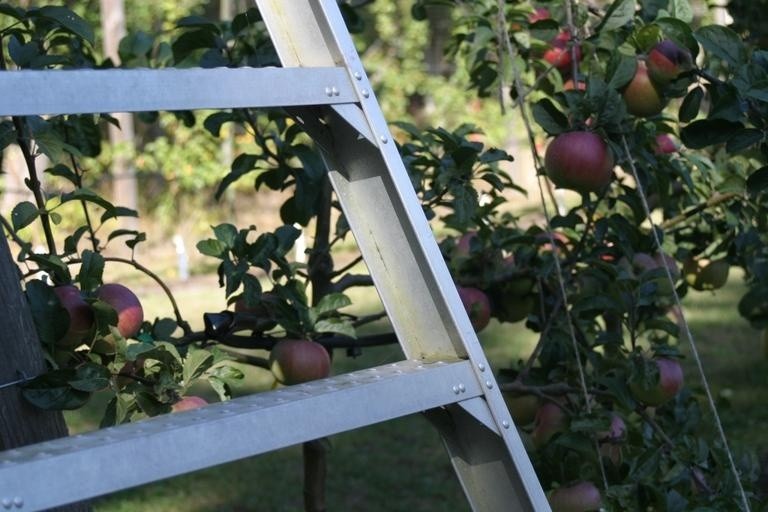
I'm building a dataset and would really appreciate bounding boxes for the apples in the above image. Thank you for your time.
[457,8,712,512]
[268,338,331,385]
[88,284,143,338]
[52,284,95,331]
[170,397,207,413]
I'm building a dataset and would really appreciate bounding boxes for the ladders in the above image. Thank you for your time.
[0,0,557,512]
